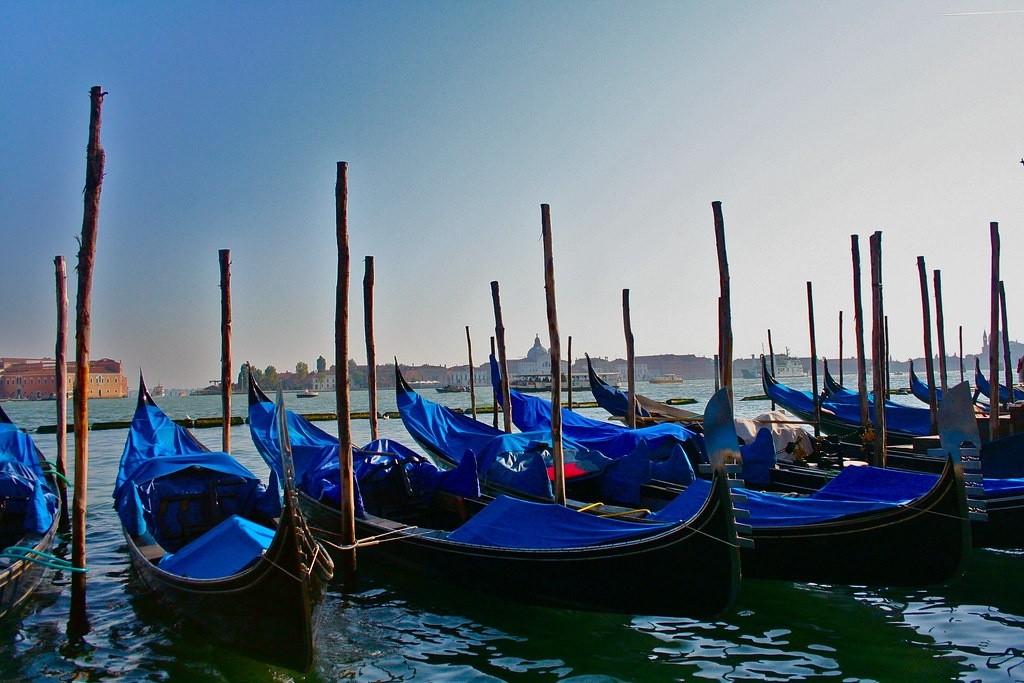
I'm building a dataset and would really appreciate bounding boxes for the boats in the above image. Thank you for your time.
[243,361,746,626]
[509,371,623,392]
[487,339,1024,555]
[393,356,970,593]
[1,404,62,628]
[435,384,471,394]
[150,385,166,398]
[112,367,339,674]
[584,348,1024,481]
[295,389,319,398]
